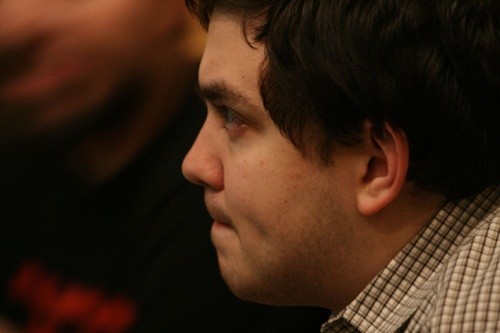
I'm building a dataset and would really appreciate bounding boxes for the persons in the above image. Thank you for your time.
[0,1,336,333]
[181,0,500,333]
